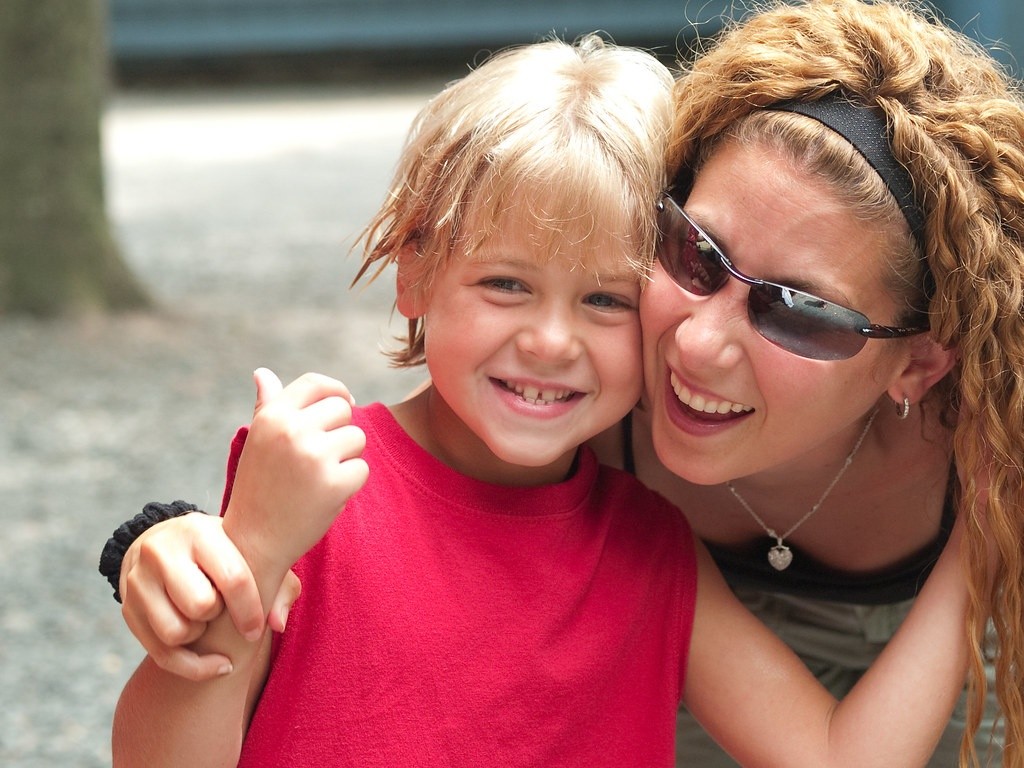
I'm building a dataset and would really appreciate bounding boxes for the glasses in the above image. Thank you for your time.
[657,171,931,360]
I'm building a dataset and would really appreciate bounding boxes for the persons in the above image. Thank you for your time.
[93,2,1024,768]
[112,28,994,767]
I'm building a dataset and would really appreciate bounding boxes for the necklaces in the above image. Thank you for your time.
[725,415,884,569]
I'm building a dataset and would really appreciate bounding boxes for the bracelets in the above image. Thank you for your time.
[98,500,207,605]
